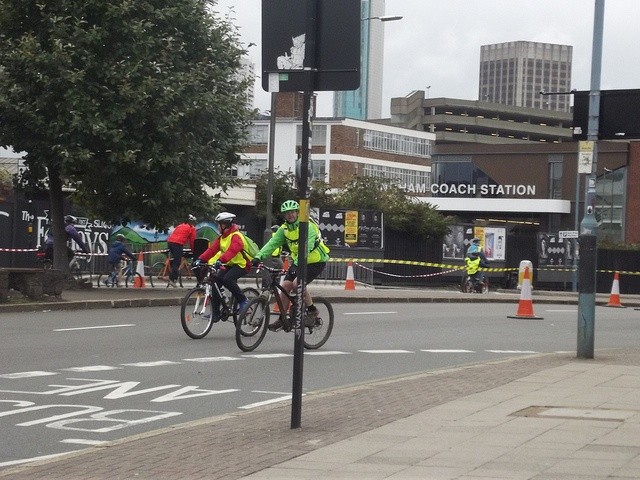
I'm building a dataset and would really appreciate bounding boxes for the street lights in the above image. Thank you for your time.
[262,16,402,290]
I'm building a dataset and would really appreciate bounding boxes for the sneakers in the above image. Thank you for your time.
[240,298,249,314]
[268,320,282,328]
[305,309,319,327]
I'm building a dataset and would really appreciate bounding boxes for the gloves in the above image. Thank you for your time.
[252,258,260,267]
[191,259,201,266]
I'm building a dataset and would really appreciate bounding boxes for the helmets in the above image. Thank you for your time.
[215,212,236,223]
[189,214,196,221]
[64,215,78,224]
[280,200,299,212]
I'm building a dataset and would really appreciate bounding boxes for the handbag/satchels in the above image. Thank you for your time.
[243,234,259,257]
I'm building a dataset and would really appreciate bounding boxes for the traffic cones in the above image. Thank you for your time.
[344,261,356,290]
[507,266,544,319]
[605,272,624,307]
[272,259,294,314]
[133,250,145,289]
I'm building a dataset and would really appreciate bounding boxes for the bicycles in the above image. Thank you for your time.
[235,259,334,352]
[97,257,143,288]
[461,261,490,294]
[181,260,264,339]
[149,250,199,289]
[35,251,91,283]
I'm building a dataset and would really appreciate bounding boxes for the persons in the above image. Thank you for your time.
[193,213,252,319]
[463,239,492,292]
[42,215,91,272]
[252,200,330,334]
[165,213,196,289]
[106,233,137,288]
